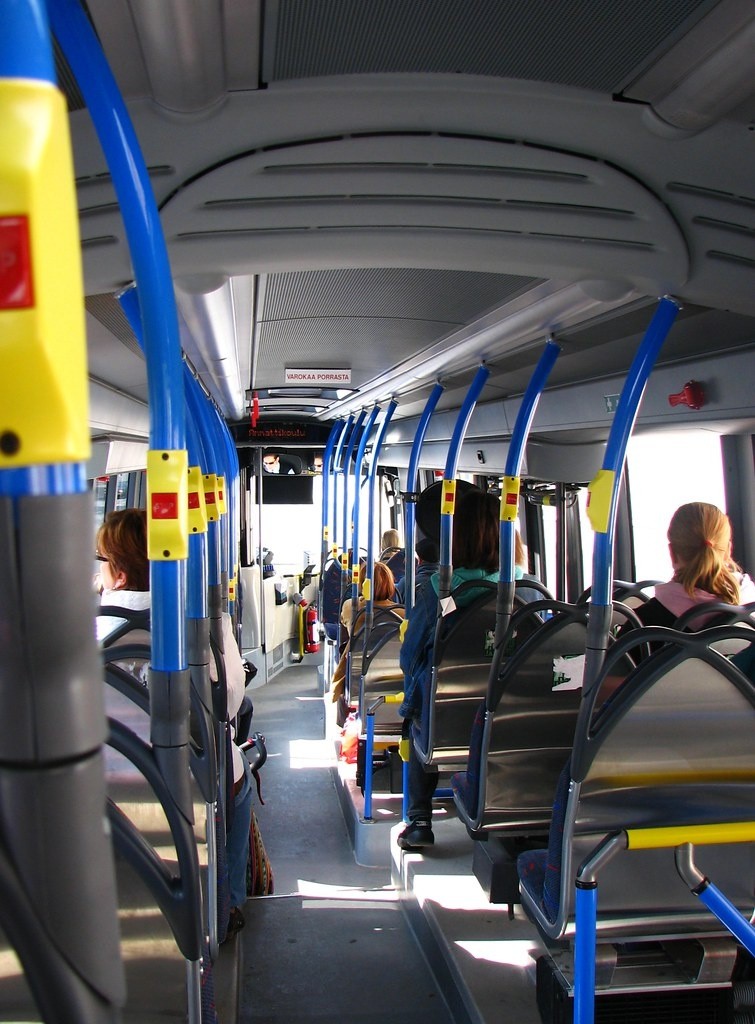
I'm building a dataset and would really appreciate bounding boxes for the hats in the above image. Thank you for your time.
[415,538,439,562]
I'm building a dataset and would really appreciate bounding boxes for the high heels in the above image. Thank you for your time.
[226,907,245,939]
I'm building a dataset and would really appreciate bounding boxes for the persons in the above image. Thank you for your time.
[595,502,755,707]
[329,481,480,703]
[95,509,253,944]
[397,494,548,850]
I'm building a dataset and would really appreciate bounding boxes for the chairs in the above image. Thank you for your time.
[315,547,755,1024]
[0,602,227,1024]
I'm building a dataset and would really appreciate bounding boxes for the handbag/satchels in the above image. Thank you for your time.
[246,806,273,897]
[242,662,257,688]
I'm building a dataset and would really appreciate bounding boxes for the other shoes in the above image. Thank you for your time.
[397,816,434,850]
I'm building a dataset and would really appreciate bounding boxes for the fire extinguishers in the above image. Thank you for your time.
[303,600,320,652]
